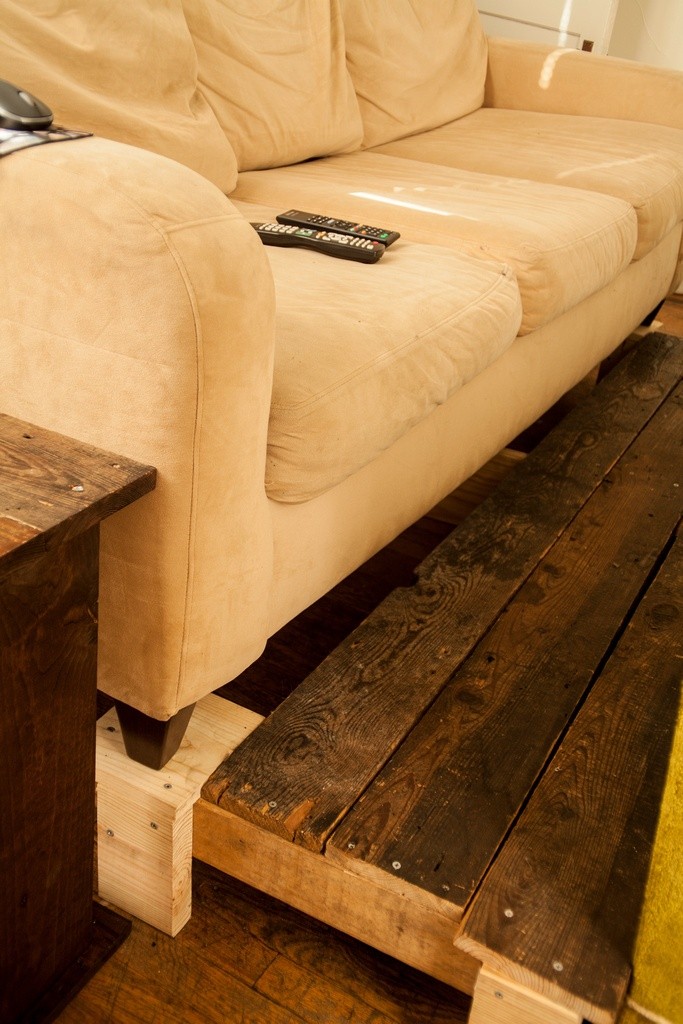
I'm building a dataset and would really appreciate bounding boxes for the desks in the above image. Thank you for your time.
[0,410,158,1024]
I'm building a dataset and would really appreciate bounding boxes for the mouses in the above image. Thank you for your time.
[0,81,54,130]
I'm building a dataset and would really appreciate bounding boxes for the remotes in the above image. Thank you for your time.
[250,210,401,265]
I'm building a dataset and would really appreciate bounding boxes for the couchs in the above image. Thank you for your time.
[0,1,683,771]
